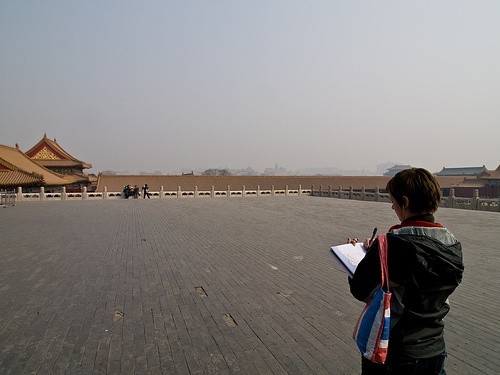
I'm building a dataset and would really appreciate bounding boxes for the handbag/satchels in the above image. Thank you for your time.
[352,233,392,365]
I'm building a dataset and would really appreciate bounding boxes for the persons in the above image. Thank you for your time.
[347,168,464,375]
[123,183,151,199]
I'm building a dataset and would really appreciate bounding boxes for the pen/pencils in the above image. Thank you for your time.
[366,227,377,251]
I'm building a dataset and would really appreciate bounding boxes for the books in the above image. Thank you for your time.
[330,242,369,279]
